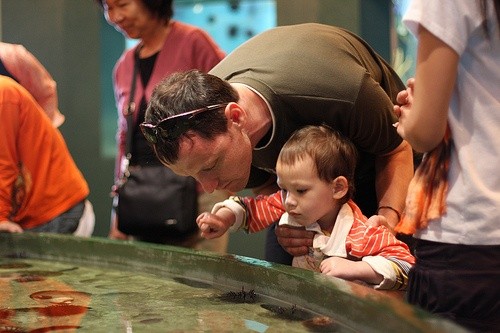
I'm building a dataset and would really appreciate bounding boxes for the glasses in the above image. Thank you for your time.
[138,103,228,143]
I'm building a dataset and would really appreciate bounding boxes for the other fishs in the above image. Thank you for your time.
[1,288,93,333]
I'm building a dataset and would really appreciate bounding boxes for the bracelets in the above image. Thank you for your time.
[377,206,401,223]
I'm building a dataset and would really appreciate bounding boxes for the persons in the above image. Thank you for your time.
[393,0,500,333]
[140,22,423,266]
[0,41,95,240]
[99,0,229,254]
[196,125,416,291]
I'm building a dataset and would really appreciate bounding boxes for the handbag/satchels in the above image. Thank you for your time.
[115,164,197,242]
